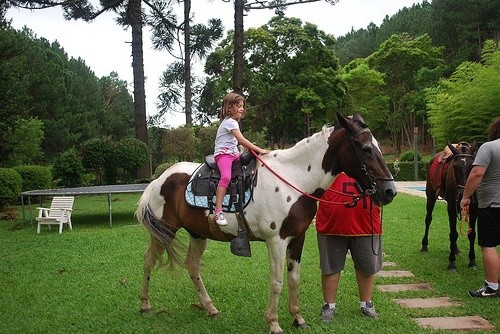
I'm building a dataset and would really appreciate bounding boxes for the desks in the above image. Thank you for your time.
[21,183,148,228]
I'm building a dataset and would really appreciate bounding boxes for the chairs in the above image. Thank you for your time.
[35,196,74,234]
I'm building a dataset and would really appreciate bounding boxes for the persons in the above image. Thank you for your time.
[460,116,500,299]
[315,171,384,323]
[214,93,270,226]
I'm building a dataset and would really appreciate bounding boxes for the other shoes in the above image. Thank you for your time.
[319,303,336,323]
[360,301,377,318]
[214,212,228,226]
[468,280,500,298]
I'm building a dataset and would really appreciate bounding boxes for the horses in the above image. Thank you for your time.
[421,139,478,272]
[134,112,397,334]
